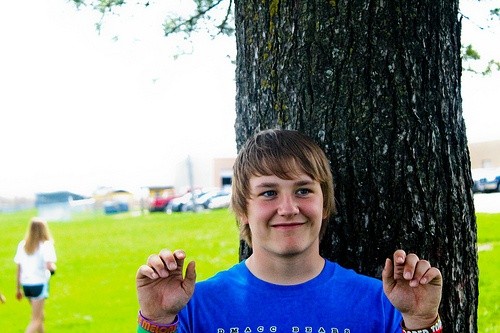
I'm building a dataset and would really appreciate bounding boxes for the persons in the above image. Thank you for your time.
[1,289,7,306]
[14,217,58,333]
[135,129,448,333]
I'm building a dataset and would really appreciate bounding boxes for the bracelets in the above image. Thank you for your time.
[400,314,443,333]
[137,326,150,333]
[139,310,181,333]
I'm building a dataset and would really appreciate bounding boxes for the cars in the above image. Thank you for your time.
[142,186,232,212]
[470,167,500,195]
